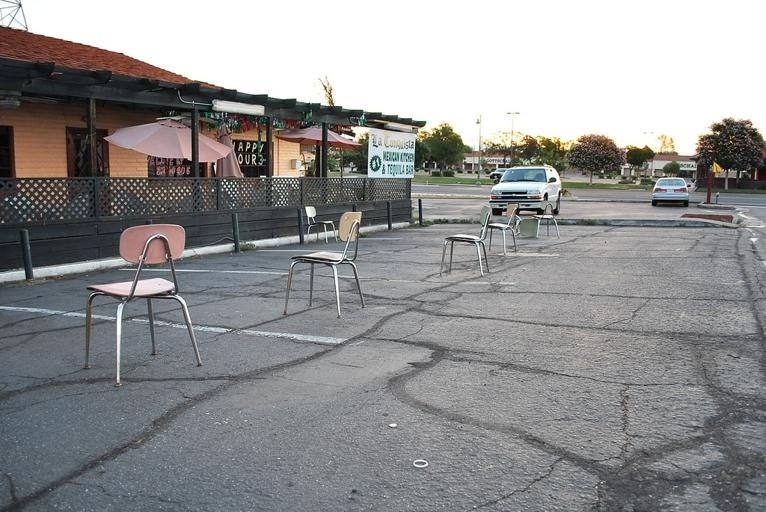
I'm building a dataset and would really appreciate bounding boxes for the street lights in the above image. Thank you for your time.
[507,112,520,163]
[476,114,482,179]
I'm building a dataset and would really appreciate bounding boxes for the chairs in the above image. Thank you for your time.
[283,205,367,318]
[87,224,201,382]
[439,201,561,278]
[511,173,544,181]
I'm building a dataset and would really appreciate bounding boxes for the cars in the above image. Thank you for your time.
[489,168,507,179]
[651,178,691,207]
[489,166,563,215]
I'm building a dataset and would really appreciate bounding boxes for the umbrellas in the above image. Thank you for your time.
[103,120,233,178]
[216,121,245,178]
[272,125,363,178]
